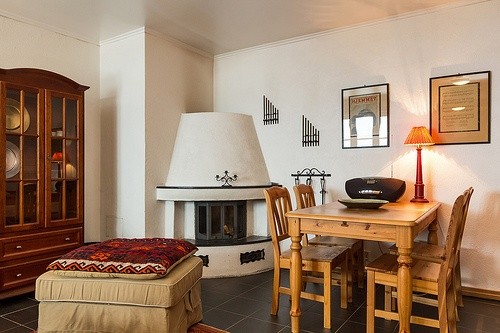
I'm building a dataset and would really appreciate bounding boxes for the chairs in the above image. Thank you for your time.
[263,185,474,333]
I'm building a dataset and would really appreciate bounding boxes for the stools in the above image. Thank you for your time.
[35,238,203,333]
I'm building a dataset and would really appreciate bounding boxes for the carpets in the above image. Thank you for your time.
[188,323,230,333]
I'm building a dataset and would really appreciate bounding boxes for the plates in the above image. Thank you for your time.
[5,97,30,134]
[6,140,20,179]
[337,198,389,209]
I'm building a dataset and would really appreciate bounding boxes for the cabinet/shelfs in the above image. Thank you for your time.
[0,67,85,300]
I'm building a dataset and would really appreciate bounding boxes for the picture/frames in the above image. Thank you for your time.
[427,70,492,146]
[342,84,395,150]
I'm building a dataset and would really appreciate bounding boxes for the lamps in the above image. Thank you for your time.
[404,126,435,203]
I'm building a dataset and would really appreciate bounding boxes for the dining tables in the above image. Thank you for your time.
[285,198,442,333]
